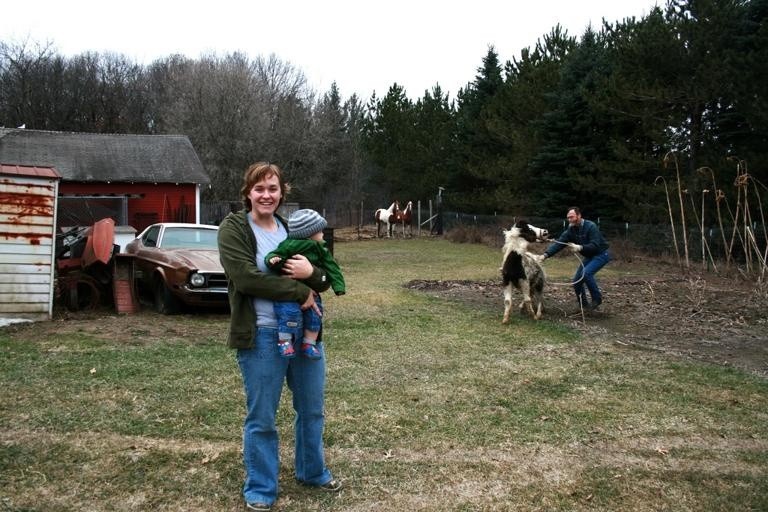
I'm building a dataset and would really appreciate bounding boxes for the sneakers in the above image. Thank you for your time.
[320,479,343,491]
[587,301,602,312]
[300,343,321,361]
[572,307,581,314]
[246,501,270,511]
[278,339,296,359]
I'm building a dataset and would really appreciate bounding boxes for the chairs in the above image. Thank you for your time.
[161,237,181,245]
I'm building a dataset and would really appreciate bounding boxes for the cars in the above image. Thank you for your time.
[125,222,233,317]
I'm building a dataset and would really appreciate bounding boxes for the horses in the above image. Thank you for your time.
[498,215,550,324]
[374,200,414,240]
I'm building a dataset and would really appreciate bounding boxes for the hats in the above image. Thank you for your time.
[287,209,327,238]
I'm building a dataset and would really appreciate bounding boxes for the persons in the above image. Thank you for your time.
[537,206,611,312]
[262,207,347,361]
[215,159,343,510]
